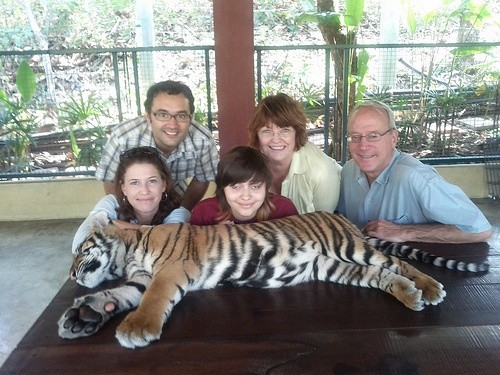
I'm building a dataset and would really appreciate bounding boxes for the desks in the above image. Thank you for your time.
[0,230,500,375]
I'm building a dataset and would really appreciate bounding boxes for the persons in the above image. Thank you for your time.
[190,145,300,226]
[94,79,220,213]
[71,146,191,255]
[334,100,493,243]
[247,93,343,217]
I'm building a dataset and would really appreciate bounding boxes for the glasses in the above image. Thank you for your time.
[120,146,164,170]
[257,127,297,139]
[148,108,192,122]
[345,129,391,143]
